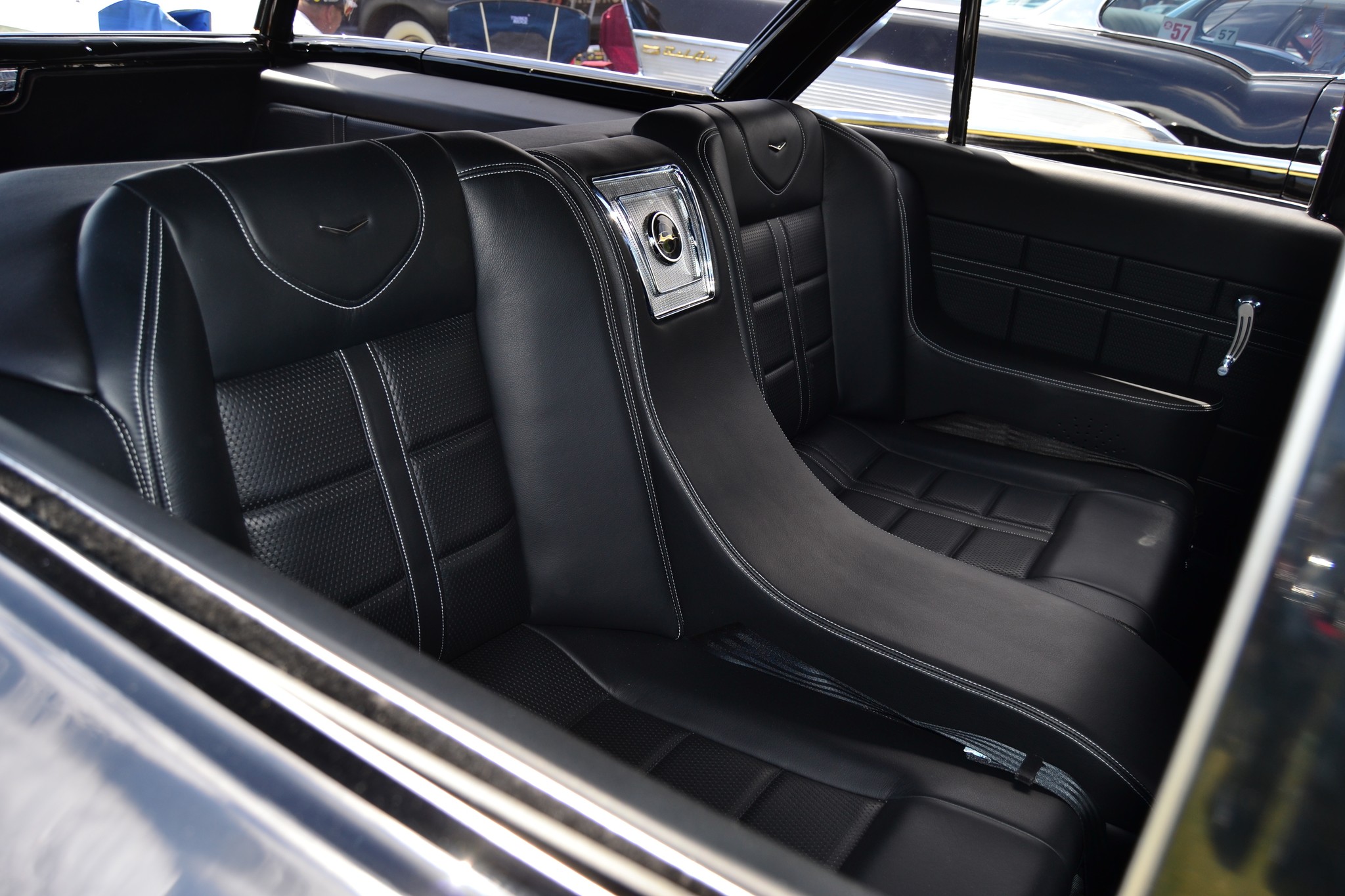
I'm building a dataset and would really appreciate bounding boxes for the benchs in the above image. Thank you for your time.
[77,91,1193,895]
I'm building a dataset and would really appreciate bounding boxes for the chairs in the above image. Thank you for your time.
[447,1,647,77]
[97,0,211,32]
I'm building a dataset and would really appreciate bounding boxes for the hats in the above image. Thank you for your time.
[305,0,359,27]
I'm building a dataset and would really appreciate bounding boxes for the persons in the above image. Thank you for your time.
[222,0,357,35]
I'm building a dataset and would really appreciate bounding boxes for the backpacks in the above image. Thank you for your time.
[626,0,666,32]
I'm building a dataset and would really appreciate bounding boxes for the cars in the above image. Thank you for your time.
[1085,0,1345,158]
[0,0,1345,896]
